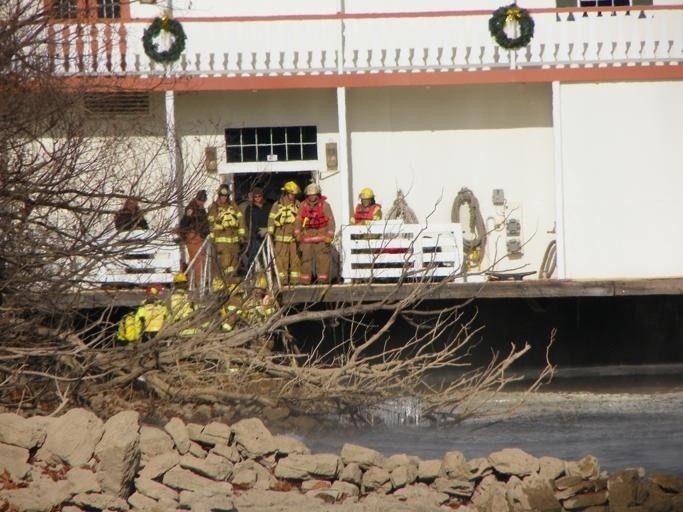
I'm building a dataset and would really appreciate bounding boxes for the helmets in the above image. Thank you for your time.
[146,283,163,297]
[196,189,209,201]
[254,277,268,290]
[215,185,233,197]
[304,182,322,196]
[210,277,226,293]
[359,187,375,200]
[280,181,302,195]
[228,283,246,296]
[171,273,188,283]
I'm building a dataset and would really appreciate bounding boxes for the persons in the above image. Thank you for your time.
[175,180,335,284]
[114,274,276,349]
[350,187,382,284]
[115,197,155,273]
